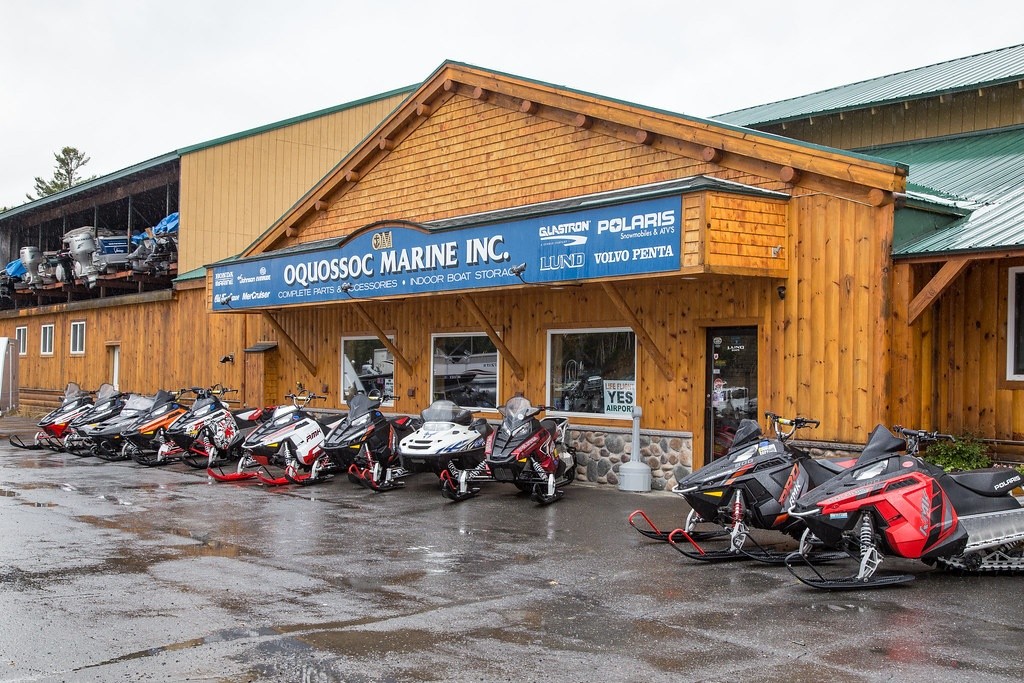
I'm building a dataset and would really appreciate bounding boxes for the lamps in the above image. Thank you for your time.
[777,285,786,299]
[220,297,283,311]
[220,354,235,363]
[509,262,584,287]
[341,284,406,302]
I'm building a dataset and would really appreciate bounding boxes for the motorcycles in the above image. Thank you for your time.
[132,384,261,469]
[441,392,578,504]
[285,389,424,488]
[629,411,861,561]
[361,400,494,491]
[563,376,603,412]
[784,424,1024,588]
[9,382,191,462]
[206,389,327,485]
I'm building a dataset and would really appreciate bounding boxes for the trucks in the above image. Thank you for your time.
[711,387,757,417]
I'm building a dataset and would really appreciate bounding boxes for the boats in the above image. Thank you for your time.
[358,339,498,400]
